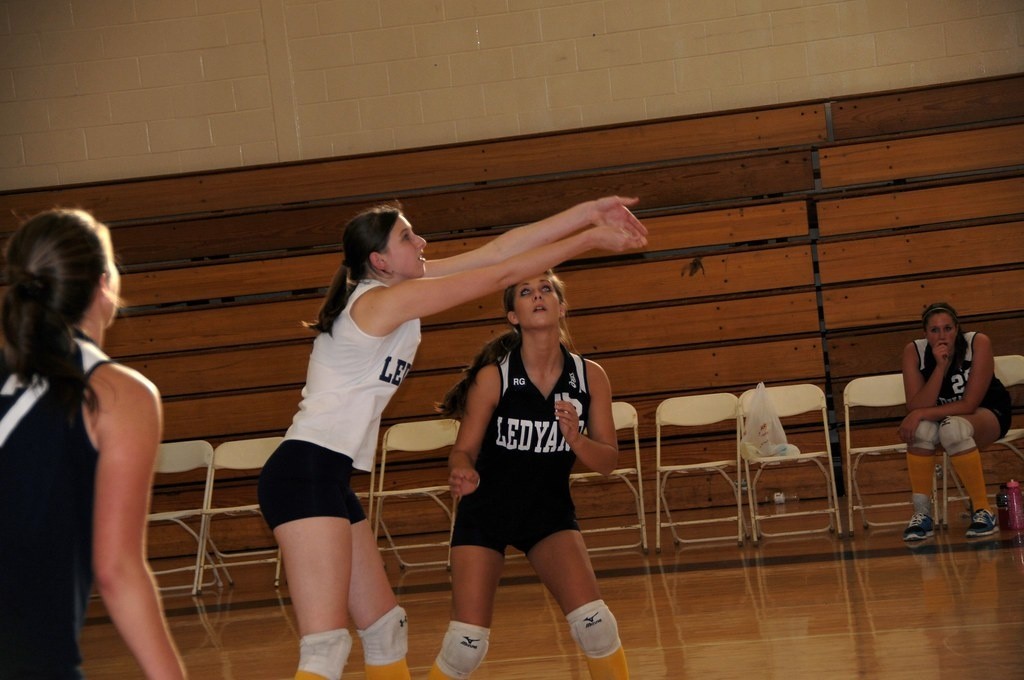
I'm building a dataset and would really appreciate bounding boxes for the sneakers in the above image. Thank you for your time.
[902,512,934,541]
[965,508,999,537]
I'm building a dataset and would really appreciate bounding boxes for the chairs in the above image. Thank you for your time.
[375,419,461,571]
[843,373,939,537]
[147,440,213,594]
[654,392,751,554]
[737,383,843,547]
[941,355,1024,532]
[568,402,648,554]
[198,437,283,600]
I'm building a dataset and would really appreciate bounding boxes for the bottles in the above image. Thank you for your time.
[996,483,1008,529]
[1007,479,1024,529]
[763,492,800,505]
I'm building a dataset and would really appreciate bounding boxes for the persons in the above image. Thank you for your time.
[427,264,637,679]
[0,203,188,680]
[897,301,1013,539]
[255,192,649,679]
